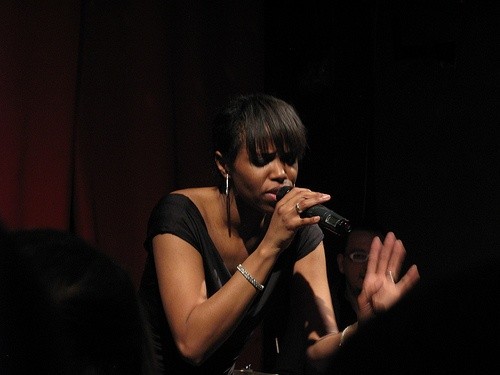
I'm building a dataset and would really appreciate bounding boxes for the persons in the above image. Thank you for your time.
[0,228,161,375]
[137,93,419,375]
[331,222,423,375]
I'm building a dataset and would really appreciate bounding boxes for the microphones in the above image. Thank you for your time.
[278,186,353,235]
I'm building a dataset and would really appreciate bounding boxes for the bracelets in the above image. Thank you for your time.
[339,325,351,350]
[235,264,265,291]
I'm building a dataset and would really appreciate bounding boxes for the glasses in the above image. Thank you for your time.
[344,251,369,263]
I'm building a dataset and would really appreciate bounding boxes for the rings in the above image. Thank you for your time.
[386,270,397,278]
[296,204,303,214]
